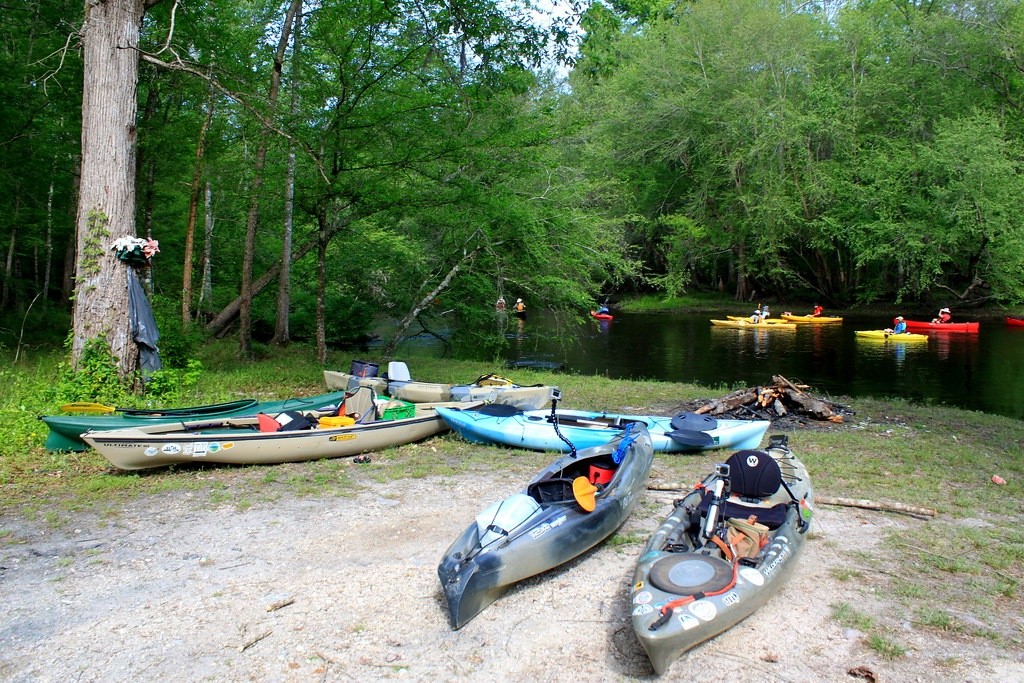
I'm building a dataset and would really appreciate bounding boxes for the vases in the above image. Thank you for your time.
[116,249,144,265]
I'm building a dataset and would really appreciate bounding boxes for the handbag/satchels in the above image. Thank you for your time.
[273,411,312,430]
[725,514,770,568]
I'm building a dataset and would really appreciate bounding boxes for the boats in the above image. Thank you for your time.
[78,401,486,471]
[591,310,613,320]
[437,422,654,629]
[780,312,843,321]
[854,330,929,340]
[894,316,979,330]
[631,435,815,676]
[514,310,527,320]
[710,316,796,329]
[37,391,348,452]
[324,361,563,411]
[435,405,771,450]
[1006,316,1024,326]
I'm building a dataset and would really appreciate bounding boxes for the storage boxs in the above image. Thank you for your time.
[319,416,354,429]
[374,394,416,420]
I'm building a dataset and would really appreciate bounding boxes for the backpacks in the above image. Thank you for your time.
[517,303,523,310]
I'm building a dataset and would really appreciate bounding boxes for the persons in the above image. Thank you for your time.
[513,298,526,311]
[884,316,906,334]
[752,310,760,324]
[813,303,821,317]
[599,304,608,314]
[497,296,505,309]
[762,306,770,319]
[932,307,951,323]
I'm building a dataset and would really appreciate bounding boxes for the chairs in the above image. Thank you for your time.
[697,448,787,523]
[387,360,414,381]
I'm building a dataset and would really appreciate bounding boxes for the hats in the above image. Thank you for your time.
[941,308,951,313]
[896,316,903,322]
[517,298,522,302]
[813,303,819,306]
[763,306,769,309]
[754,310,761,314]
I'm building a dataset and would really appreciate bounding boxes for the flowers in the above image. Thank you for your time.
[111,236,161,257]
